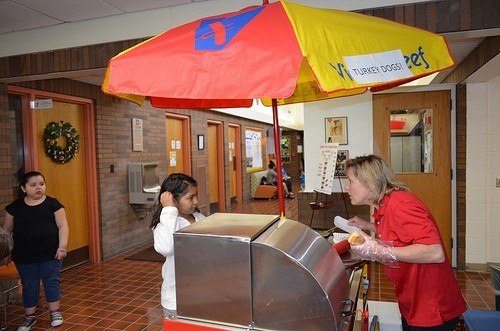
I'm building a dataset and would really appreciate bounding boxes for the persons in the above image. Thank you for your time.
[344,154,468,331]
[266,160,295,199]
[5,170,69,331]
[150,172,208,319]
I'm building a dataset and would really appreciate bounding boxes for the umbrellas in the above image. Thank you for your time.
[101,0,455,217]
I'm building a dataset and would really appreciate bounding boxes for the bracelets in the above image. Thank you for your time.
[57,248,66,253]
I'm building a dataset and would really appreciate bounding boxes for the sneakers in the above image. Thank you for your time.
[17,313,37,331]
[49,309,63,326]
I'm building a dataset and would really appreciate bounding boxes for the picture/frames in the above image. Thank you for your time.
[334,150,349,179]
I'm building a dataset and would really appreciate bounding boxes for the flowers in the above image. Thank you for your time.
[43,121,80,165]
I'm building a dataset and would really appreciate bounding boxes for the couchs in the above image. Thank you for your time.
[250,170,278,201]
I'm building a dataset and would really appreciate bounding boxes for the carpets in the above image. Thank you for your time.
[123,246,166,262]
